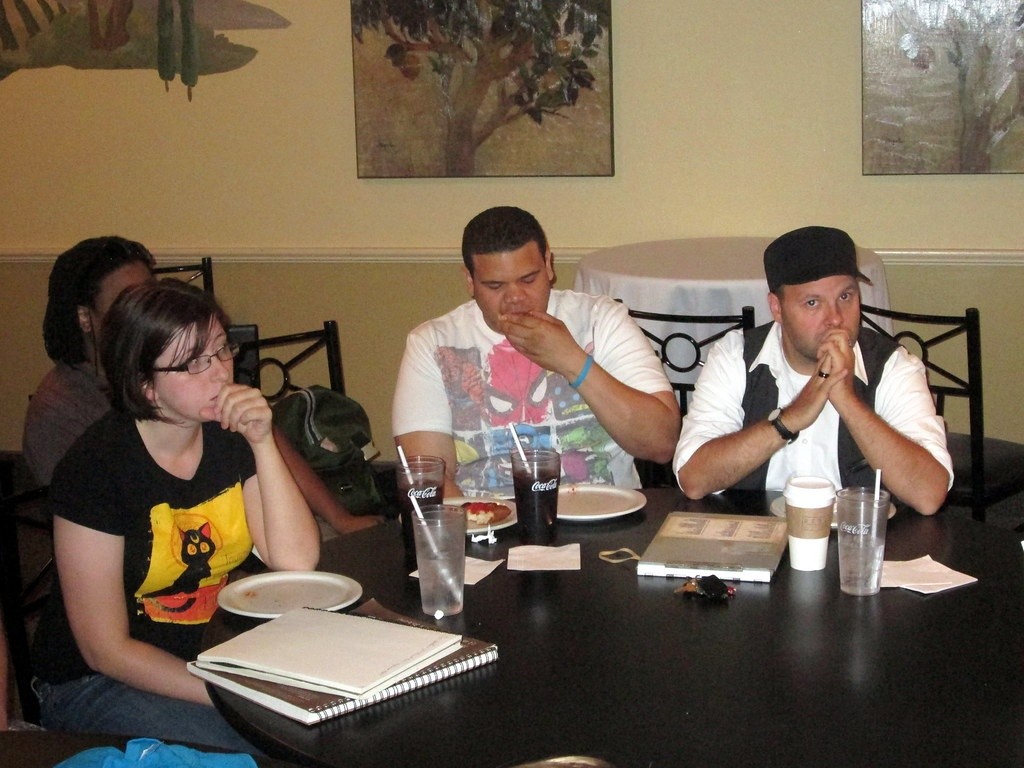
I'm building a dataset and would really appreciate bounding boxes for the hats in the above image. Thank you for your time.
[763,226,872,295]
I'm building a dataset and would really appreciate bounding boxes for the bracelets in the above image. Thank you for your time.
[569,354,594,388]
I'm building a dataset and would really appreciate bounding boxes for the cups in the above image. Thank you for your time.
[396,456,445,552]
[783,475,835,572]
[836,487,891,597]
[411,505,467,616]
[510,450,561,546]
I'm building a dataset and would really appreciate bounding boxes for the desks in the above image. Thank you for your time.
[572,236,895,413]
[200,430,1024,768]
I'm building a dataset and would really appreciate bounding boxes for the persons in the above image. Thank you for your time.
[391,205,682,500]
[673,227,954,515]
[30,277,320,752]
[18,237,385,640]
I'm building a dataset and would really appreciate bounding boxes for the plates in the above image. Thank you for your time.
[398,496,518,536]
[218,570,363,619]
[769,495,897,528]
[556,483,647,522]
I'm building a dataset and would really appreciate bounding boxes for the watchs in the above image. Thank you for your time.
[768,407,799,444]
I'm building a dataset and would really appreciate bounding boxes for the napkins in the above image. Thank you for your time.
[901,560,978,595]
[880,552,952,588]
[409,555,505,585]
[507,542,581,571]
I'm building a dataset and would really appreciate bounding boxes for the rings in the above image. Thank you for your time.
[818,371,829,378]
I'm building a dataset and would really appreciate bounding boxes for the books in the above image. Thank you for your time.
[637,512,790,583]
[186,599,499,724]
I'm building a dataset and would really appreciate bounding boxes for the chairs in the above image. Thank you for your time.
[859,303,985,437]
[614,300,755,419]
[0,449,56,726]
[232,320,399,519]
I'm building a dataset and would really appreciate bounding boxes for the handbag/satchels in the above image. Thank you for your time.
[269,383,389,516]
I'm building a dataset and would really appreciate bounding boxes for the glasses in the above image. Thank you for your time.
[153,340,241,374]
